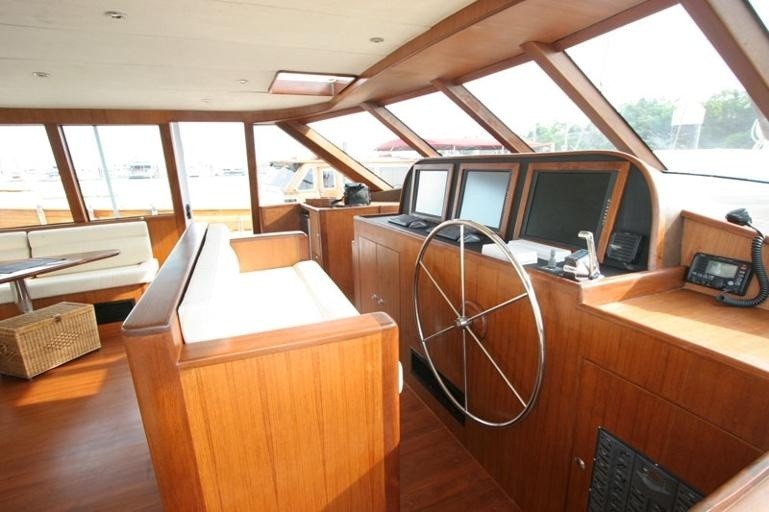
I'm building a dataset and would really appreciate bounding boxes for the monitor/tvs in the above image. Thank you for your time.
[455,168,513,232]
[409,164,455,223]
[517,162,618,255]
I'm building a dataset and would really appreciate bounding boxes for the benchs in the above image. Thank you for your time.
[18,221,162,322]
[118,217,405,512]
[0,226,35,324]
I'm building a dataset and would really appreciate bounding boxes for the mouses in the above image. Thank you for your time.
[457,233,485,245]
[409,221,428,229]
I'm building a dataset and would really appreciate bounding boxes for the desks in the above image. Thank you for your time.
[0,248,122,314]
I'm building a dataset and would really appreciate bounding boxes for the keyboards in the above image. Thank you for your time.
[388,214,419,226]
[425,223,477,241]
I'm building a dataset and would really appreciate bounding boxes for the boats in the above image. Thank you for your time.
[0,160,248,192]
[264,156,425,209]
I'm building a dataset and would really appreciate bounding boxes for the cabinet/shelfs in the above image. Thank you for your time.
[353,234,411,377]
[549,286,769,512]
[308,202,400,307]
